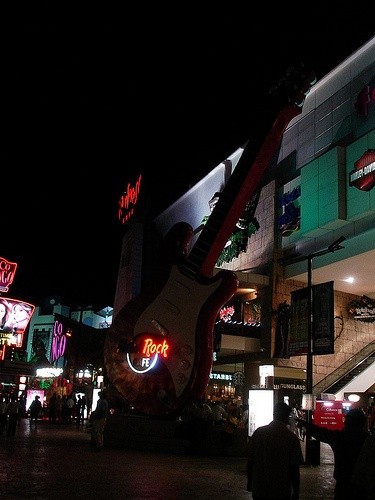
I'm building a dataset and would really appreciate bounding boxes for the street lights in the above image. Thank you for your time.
[277,235,348,465]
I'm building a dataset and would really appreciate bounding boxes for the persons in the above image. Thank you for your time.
[201,399,249,430]
[1,394,26,435]
[294,407,375,499]
[48,393,87,425]
[29,396,41,429]
[91,392,108,448]
[247,403,300,499]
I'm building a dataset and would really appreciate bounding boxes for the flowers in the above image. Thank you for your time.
[347,294,375,323]
[219,306,235,324]
